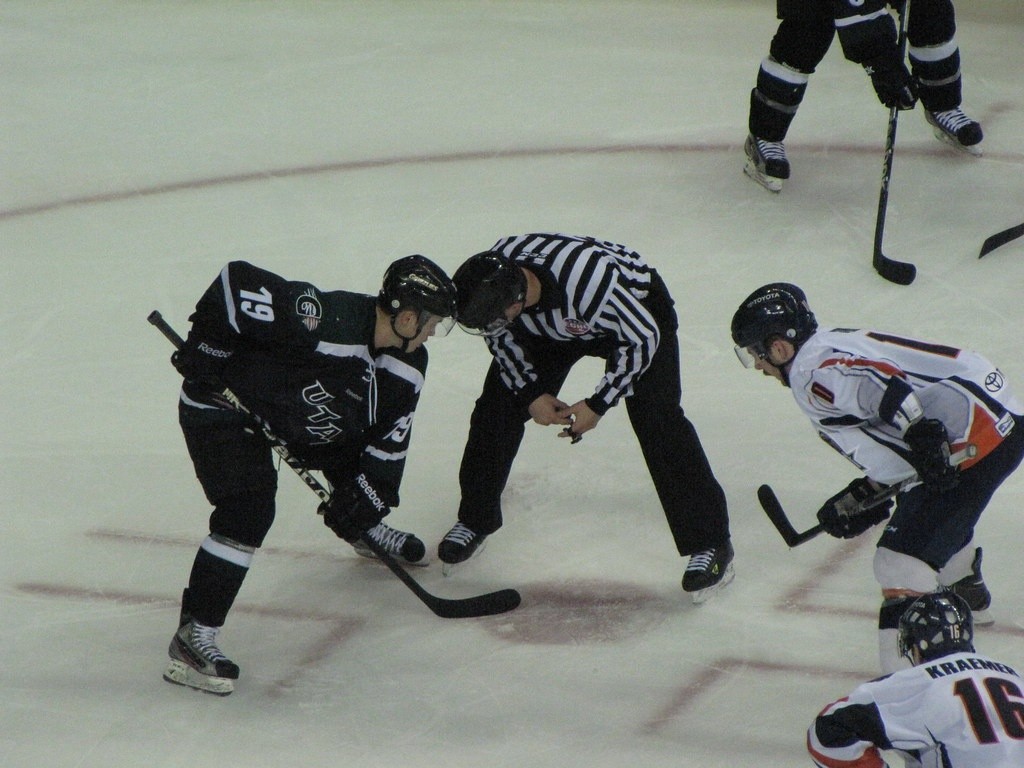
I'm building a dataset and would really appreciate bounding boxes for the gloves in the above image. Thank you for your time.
[904,419,970,488]
[816,475,894,539]
[169,311,248,394]
[317,463,400,544]
[860,49,922,110]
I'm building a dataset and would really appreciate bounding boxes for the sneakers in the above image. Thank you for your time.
[354,520,430,567]
[919,104,982,158]
[163,619,240,694]
[437,521,486,576]
[943,546,992,627]
[741,135,790,193]
[682,537,735,603]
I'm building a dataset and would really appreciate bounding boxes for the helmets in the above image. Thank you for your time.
[451,250,527,335]
[730,282,818,348]
[379,254,456,318]
[896,589,976,662]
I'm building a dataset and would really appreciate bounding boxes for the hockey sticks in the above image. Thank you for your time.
[871,0,919,286]
[976,223,1024,261]
[756,442,978,550]
[145,309,523,619]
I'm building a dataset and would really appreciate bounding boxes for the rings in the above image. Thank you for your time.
[566,427,583,445]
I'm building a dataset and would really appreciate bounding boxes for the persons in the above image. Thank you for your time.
[162,254,459,693]
[437,232,735,605]
[730,281,1024,673]
[805,588,1024,768]
[741,0,984,193]
